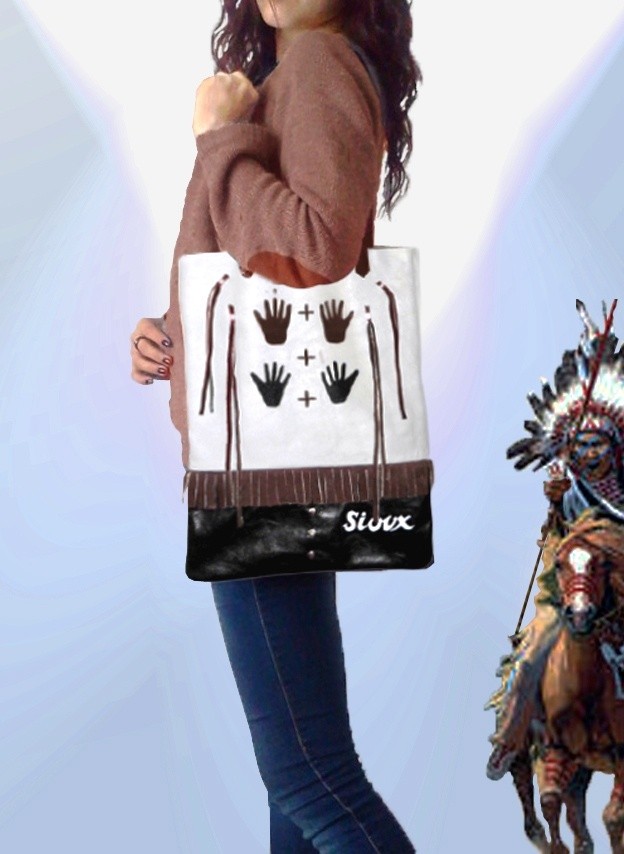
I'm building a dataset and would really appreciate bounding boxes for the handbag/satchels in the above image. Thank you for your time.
[185,40,435,582]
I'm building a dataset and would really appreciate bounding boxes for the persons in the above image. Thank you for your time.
[130,0,415,854]
[544,432,624,536]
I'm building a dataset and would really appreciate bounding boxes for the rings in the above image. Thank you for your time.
[134,335,145,350]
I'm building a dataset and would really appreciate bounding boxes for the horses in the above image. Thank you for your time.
[505,537,624,854]
